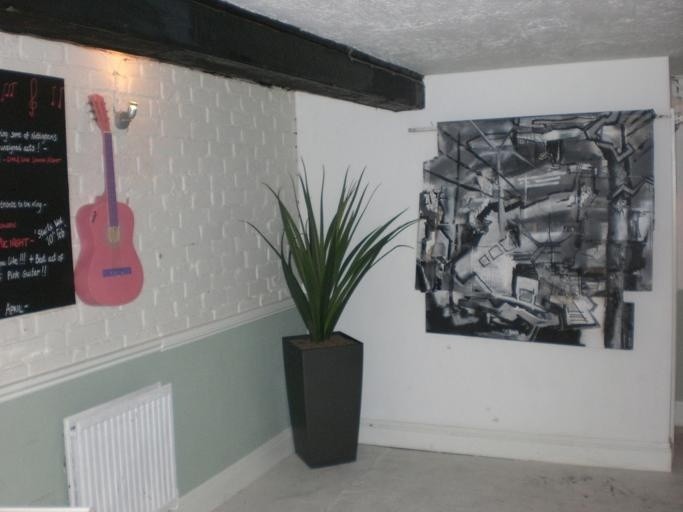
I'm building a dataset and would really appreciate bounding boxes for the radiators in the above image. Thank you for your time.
[62,382,180,512]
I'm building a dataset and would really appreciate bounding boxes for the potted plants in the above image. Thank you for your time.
[238,157,421,469]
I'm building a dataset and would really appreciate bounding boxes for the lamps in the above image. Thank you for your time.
[114,99,138,130]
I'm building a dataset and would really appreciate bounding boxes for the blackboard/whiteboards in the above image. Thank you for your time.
[0,69,76,320]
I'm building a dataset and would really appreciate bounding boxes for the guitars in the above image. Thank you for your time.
[74,94,144,306]
